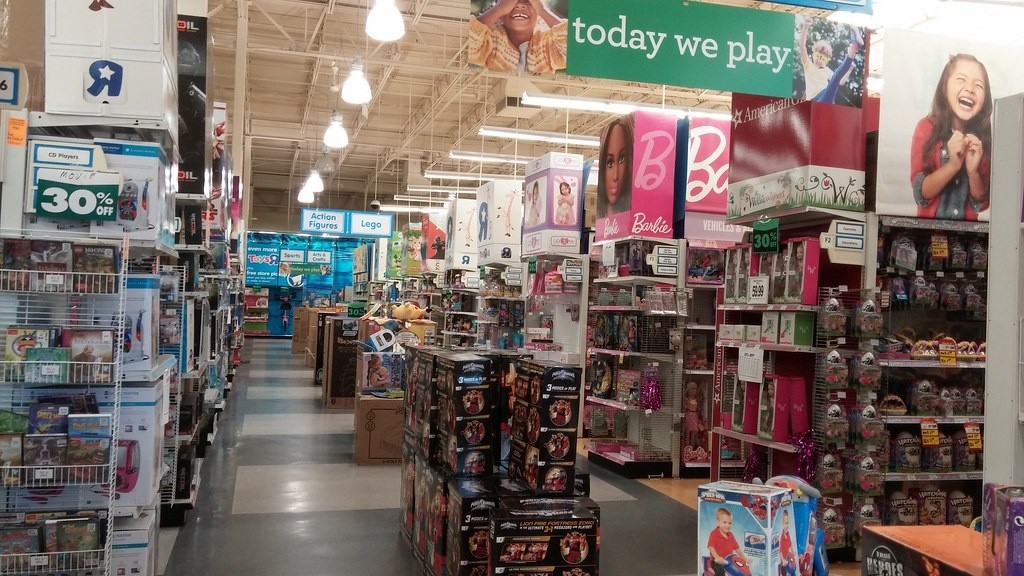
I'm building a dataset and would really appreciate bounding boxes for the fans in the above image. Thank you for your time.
[328,60,346,92]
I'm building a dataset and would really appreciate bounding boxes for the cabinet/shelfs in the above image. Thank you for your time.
[197,198,243,460]
[159,193,209,528]
[1,2,179,576]
[351,210,1024,576]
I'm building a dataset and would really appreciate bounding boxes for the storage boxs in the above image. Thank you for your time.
[982,483,1024,576]
[292,237,819,576]
[859,524,983,576]
[696,481,800,576]
[0,134,227,576]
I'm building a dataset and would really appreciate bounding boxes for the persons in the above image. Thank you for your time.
[707,508,745,575]
[798,15,859,106]
[594,112,634,219]
[368,356,391,389]
[524,178,575,227]
[910,52,992,222]
[778,509,798,575]
[70,344,102,381]
[762,242,804,295]
[467,0,568,75]
[682,378,776,450]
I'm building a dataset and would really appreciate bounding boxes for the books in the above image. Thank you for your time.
[0,236,119,573]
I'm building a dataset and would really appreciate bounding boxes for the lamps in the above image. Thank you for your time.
[304,144,324,192]
[379,91,731,215]
[323,92,349,148]
[315,146,334,173]
[297,153,314,204]
[342,8,373,104]
